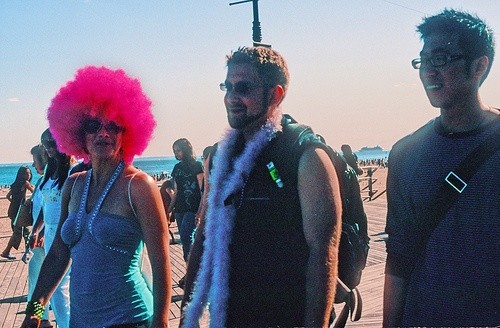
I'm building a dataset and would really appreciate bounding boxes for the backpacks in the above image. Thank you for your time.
[280,113,370,327]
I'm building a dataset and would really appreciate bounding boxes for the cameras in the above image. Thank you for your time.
[22,249,34,264]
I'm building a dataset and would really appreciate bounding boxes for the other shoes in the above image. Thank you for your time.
[0,250,17,259]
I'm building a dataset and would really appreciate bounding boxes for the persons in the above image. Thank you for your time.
[153,171,171,181]
[170,137,204,286]
[200,145,212,168]
[382,6,500,328]
[358,157,388,168]
[19,65,172,328]
[341,144,364,176]
[0,126,78,328]
[160,177,177,217]
[178,46,343,328]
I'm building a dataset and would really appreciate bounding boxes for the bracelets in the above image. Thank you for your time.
[24,302,45,320]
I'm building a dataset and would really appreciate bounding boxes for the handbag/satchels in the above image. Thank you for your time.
[13,198,33,227]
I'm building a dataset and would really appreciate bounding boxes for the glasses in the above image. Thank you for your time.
[84,115,123,136]
[412,54,471,69]
[201,155,205,160]
[219,81,266,93]
